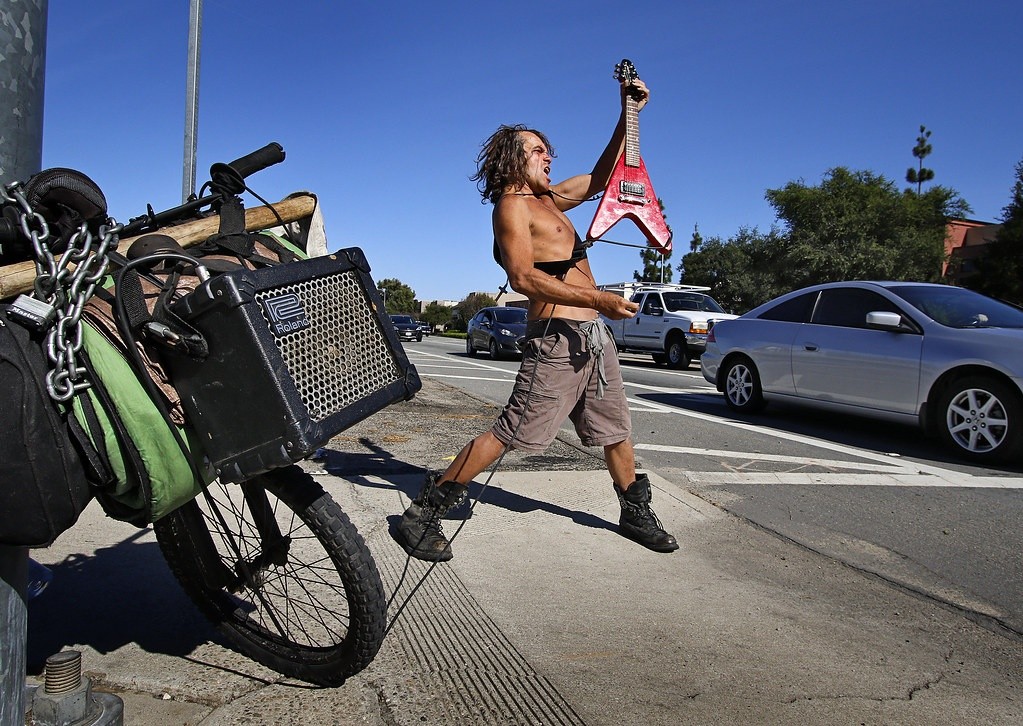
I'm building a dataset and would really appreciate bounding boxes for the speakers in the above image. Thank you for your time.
[161,247,421,484]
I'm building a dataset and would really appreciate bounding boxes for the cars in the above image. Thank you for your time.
[697,279,1023,473]
[388,313,432,342]
[465,305,528,362]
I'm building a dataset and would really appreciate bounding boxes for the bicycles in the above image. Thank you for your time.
[0,141,426,688]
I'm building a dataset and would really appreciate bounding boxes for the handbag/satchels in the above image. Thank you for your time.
[0,274,220,546]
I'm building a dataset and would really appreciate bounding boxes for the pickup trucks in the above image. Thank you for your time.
[598,284,743,371]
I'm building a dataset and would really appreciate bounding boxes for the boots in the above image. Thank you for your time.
[611,472,679,552]
[398,471,471,561]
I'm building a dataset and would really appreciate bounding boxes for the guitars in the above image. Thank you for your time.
[585,58,674,256]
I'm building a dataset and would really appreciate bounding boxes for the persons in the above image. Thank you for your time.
[395,79,681,560]
